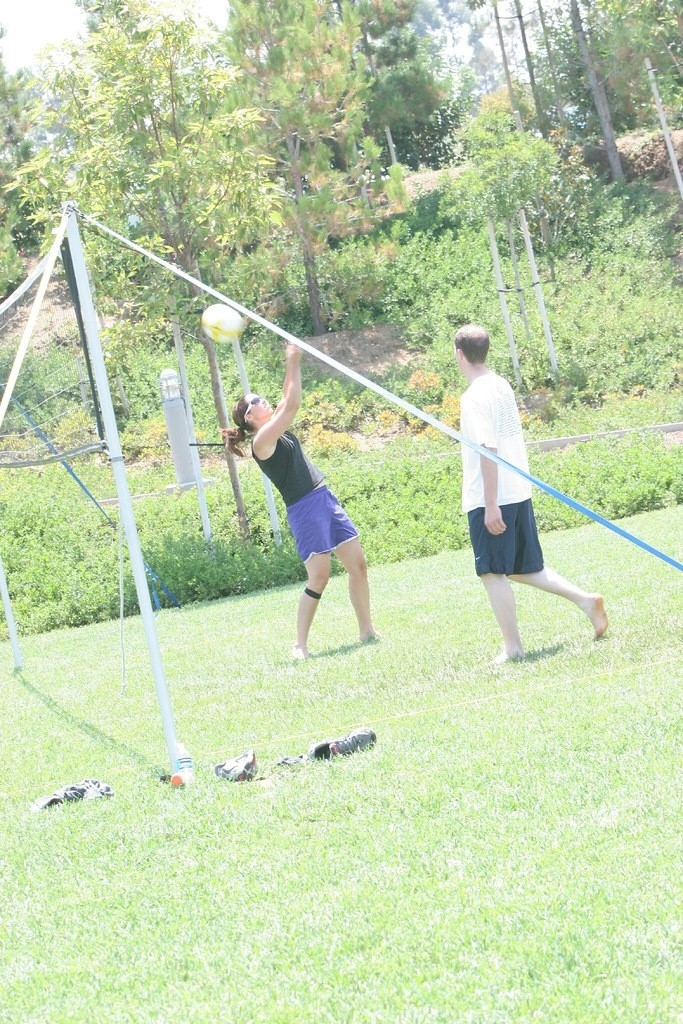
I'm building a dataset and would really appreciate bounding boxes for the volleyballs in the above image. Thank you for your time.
[201,303,243,344]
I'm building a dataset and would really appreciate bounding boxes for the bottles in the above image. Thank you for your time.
[174,743,193,783]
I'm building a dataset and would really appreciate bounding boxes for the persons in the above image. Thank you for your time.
[222,345,383,658]
[452,324,608,663]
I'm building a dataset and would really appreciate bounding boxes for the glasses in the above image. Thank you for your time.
[244,396,263,423]
